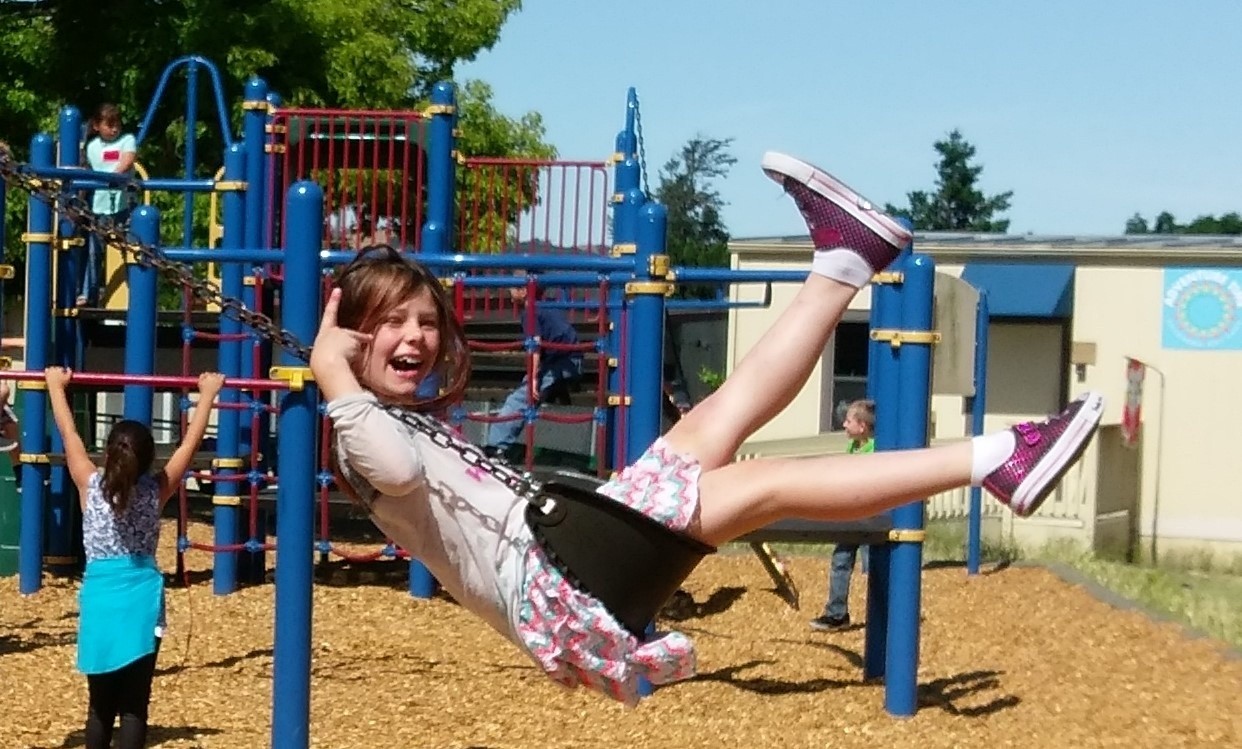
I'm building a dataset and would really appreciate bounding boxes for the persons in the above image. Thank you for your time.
[309,148,1107,708]
[47,365,227,749]
[75,102,137,308]
[481,268,578,458]
[808,400,878,632]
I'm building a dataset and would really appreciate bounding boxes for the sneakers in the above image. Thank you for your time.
[983,392,1105,516]
[808,612,850,632]
[759,151,913,273]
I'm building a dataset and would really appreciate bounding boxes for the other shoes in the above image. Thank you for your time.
[76,293,105,306]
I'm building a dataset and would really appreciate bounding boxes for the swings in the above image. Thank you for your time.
[0,161,720,648]
